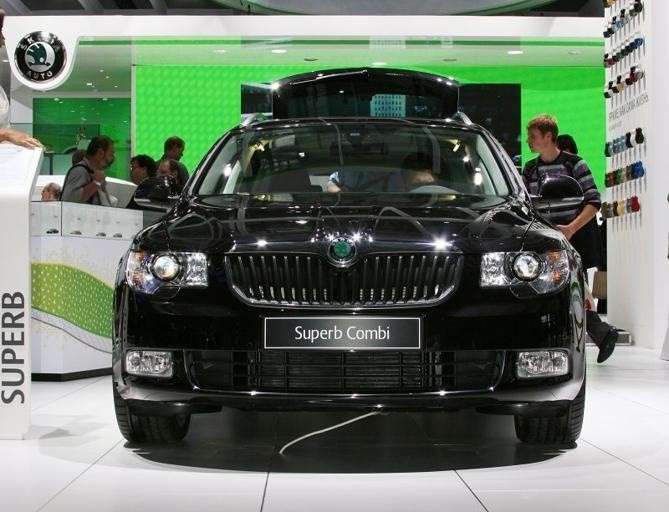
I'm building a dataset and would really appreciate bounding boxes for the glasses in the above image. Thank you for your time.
[130,166,140,172]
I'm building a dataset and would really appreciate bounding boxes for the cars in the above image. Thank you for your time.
[110,66,590,453]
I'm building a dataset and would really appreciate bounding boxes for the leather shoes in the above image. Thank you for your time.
[597,329,619,363]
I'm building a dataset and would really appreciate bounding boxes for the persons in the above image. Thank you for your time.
[40,135,190,210]
[326,164,404,192]
[401,154,437,191]
[522,115,618,363]
[0,8,44,150]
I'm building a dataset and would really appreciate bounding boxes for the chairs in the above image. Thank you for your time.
[249,144,451,198]
